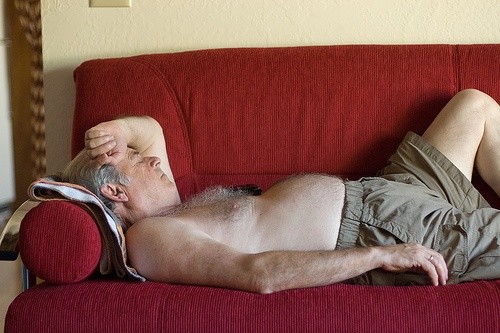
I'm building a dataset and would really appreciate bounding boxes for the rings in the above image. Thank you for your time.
[428,255,434,261]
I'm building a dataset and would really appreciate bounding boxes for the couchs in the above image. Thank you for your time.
[0,44,500,333]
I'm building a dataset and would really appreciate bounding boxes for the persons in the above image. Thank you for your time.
[59,88,500,295]
